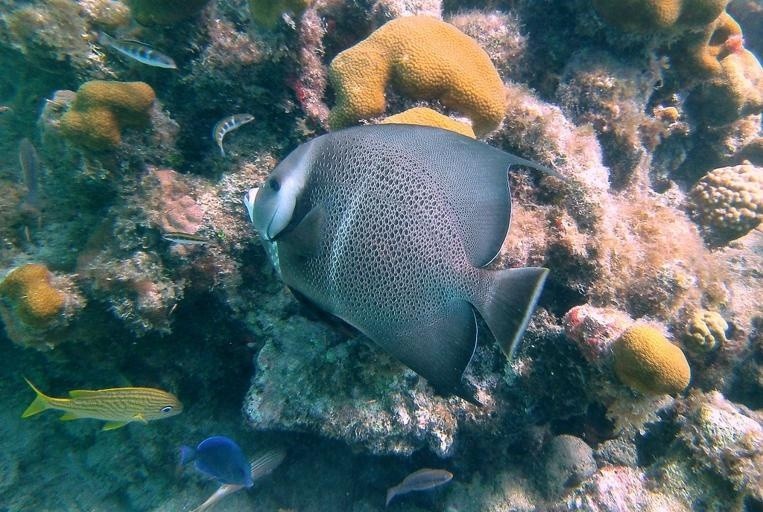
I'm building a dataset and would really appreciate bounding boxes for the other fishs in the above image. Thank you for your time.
[238,116,551,414]
